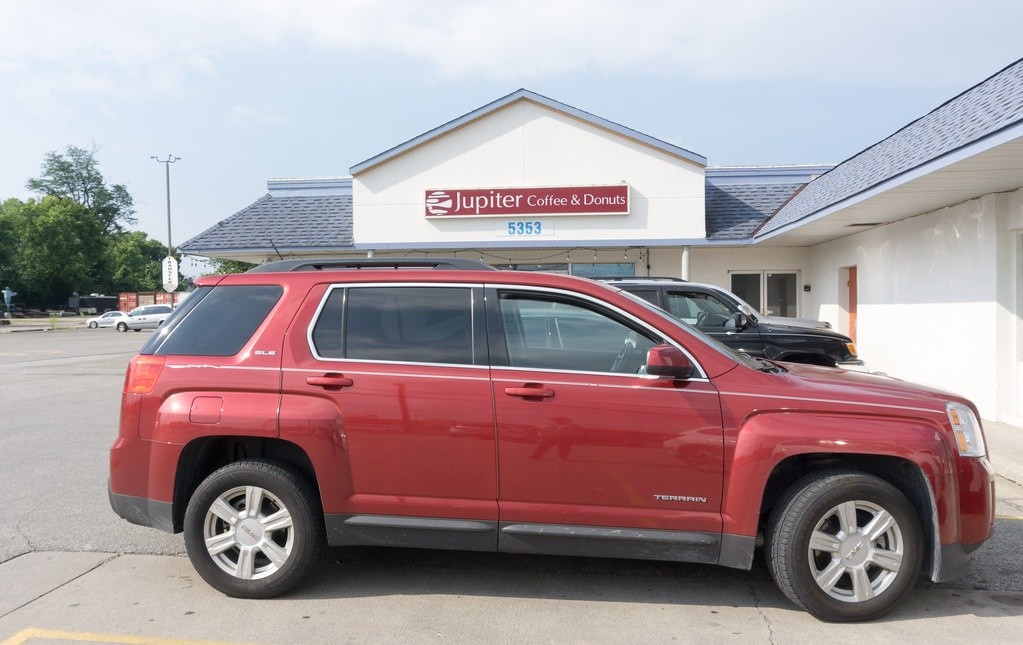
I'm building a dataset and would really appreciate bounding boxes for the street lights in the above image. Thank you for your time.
[149,154,181,313]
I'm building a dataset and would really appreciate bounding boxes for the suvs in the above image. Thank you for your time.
[112,303,177,333]
[107,258,995,624]
[412,274,871,379]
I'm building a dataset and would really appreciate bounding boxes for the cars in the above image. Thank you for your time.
[85,311,128,329]
